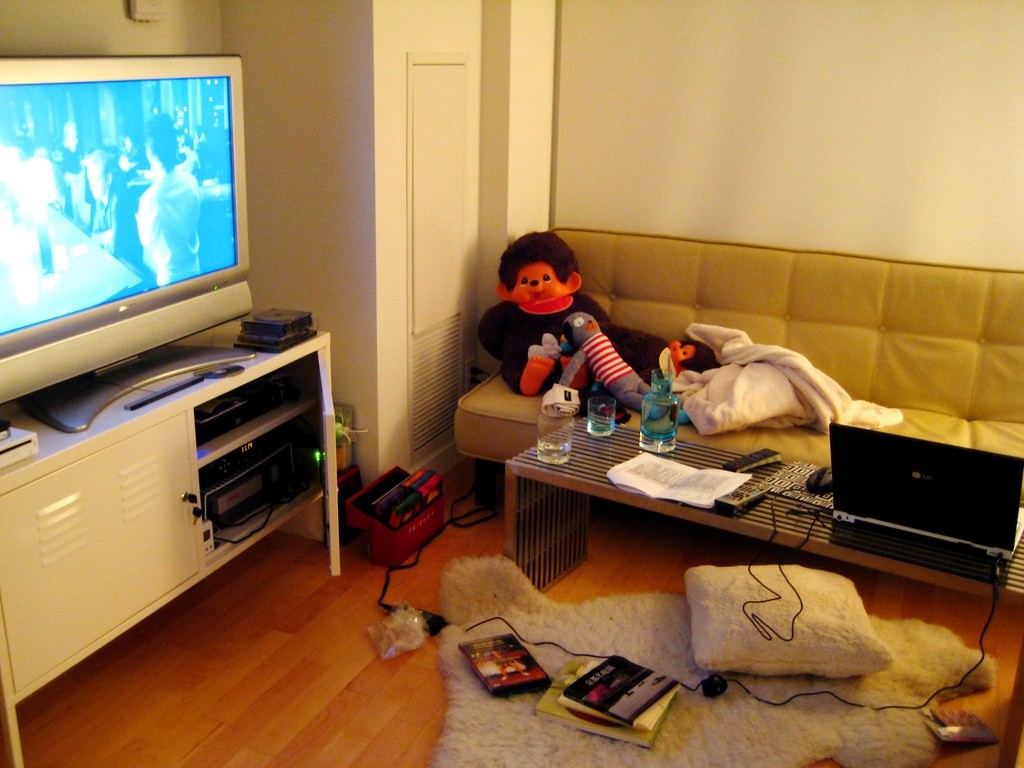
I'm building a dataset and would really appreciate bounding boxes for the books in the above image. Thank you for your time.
[534,656,682,749]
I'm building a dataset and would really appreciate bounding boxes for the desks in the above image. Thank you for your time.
[501,418,1024,768]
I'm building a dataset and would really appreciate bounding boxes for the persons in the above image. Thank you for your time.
[137,115,201,287]
[88,150,127,259]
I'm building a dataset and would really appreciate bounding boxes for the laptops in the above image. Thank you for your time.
[829,422,1024,561]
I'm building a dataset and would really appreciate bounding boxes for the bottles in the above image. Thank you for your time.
[639,369,681,453]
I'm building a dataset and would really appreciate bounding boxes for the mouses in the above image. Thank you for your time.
[806,465,834,495]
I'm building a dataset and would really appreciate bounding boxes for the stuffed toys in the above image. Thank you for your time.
[478,232,720,426]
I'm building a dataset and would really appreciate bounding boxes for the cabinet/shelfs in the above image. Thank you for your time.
[0,320,342,768]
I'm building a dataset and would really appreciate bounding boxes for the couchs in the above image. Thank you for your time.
[452,226,1024,510]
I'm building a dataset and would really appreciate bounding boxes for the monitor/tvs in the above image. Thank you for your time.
[0,54,256,433]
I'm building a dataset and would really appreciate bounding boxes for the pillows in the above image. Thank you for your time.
[682,563,894,679]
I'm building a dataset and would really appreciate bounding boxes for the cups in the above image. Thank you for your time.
[587,396,616,436]
[536,410,574,465]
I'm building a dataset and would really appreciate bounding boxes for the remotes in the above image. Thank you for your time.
[713,487,766,510]
[721,447,783,473]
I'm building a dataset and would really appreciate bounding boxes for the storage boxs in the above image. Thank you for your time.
[347,464,448,568]
